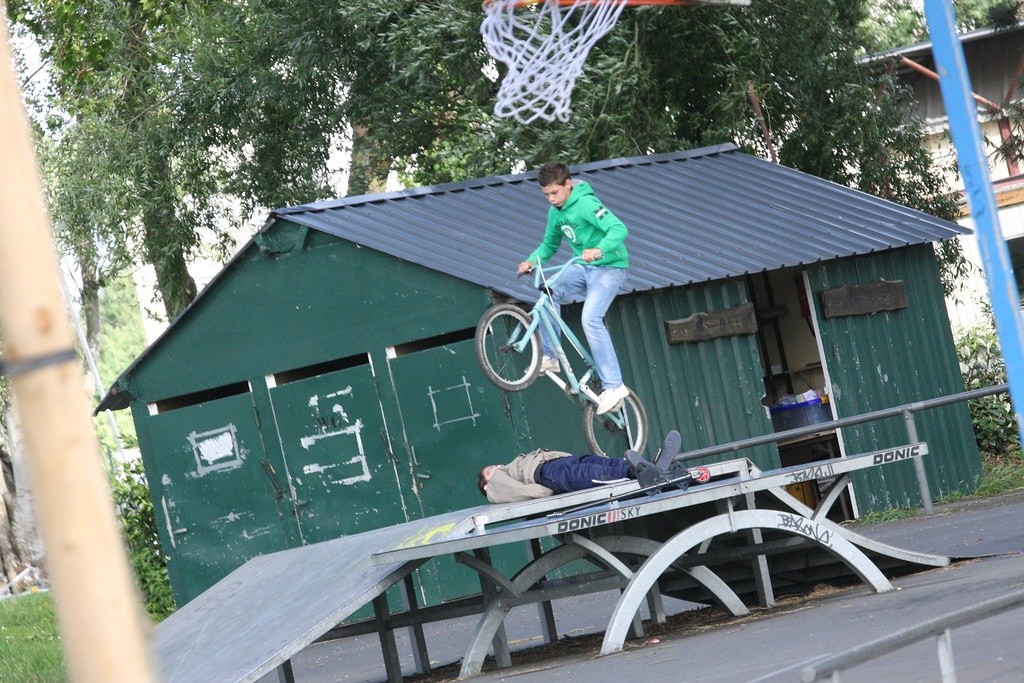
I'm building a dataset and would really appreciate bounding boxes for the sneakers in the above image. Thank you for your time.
[596,383,629,415]
[524,357,561,376]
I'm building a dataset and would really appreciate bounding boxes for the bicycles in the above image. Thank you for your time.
[475,253,648,463]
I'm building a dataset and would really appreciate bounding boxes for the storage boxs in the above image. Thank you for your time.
[767,398,833,432]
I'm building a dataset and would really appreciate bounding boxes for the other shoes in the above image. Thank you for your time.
[623,450,662,474]
[651,430,681,472]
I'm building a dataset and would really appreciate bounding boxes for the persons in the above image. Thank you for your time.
[478,430,681,503]
[519,162,630,415]
